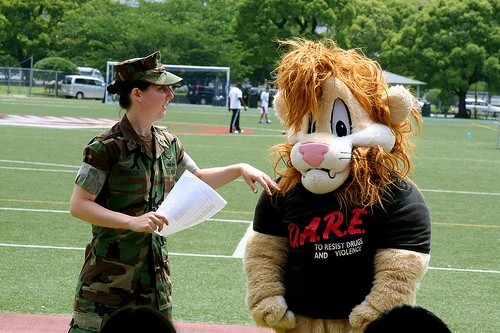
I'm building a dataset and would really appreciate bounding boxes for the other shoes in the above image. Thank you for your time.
[234,131,239,134]
[266,120,272,123]
[241,130,244,133]
[258,120,263,123]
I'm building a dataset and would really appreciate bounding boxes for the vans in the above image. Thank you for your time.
[76,67,104,80]
[61,74,116,101]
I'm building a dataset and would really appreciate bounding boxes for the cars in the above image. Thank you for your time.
[245,87,277,105]
[46,80,63,90]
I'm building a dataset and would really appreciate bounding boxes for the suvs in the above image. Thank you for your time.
[453,99,499,117]
[188,81,233,105]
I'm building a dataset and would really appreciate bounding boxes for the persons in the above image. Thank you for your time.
[67,51,280,333]
[228,83,248,135]
[363,304,452,333]
[100,306,177,333]
[259,87,272,123]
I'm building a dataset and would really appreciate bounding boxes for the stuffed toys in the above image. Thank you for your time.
[242,35,432,333]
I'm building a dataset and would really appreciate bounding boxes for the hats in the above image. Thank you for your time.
[113,51,183,87]
